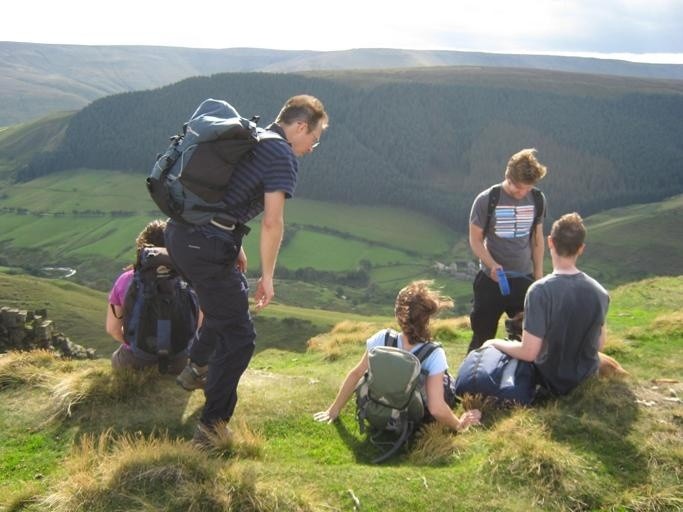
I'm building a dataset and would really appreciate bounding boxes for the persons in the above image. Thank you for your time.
[313,279,481,433]
[467,147,547,354]
[478,211,611,394]
[162,94,329,447]
[106,219,204,373]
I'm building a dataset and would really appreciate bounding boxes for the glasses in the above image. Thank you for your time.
[297,121,321,148]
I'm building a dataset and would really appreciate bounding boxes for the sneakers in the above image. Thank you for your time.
[177,363,209,392]
[193,423,234,447]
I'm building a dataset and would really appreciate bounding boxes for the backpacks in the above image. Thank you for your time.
[145,97,287,226]
[354,328,441,464]
[455,341,539,407]
[110,246,201,361]
